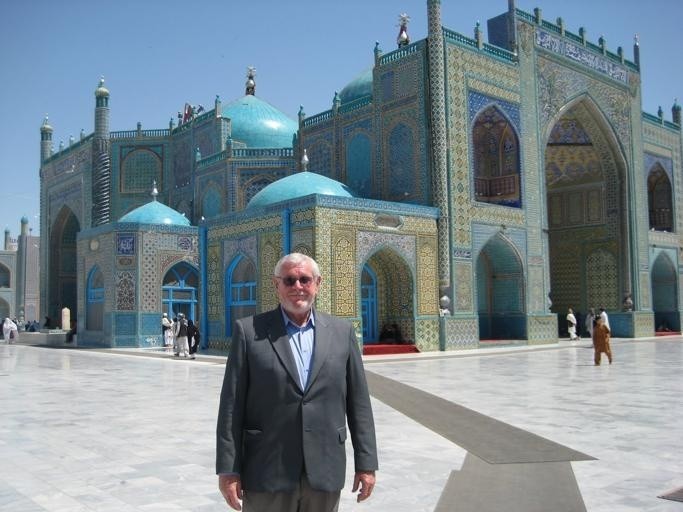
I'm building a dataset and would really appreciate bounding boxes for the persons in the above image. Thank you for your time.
[0,315,50,344]
[574,312,583,339]
[64,319,76,344]
[161,311,200,360]
[657,319,668,331]
[592,314,612,366]
[599,307,610,332]
[213,251,379,512]
[565,307,578,339]
[377,322,403,345]
[584,307,596,339]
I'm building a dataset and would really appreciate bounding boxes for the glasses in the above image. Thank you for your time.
[277,276,312,287]
